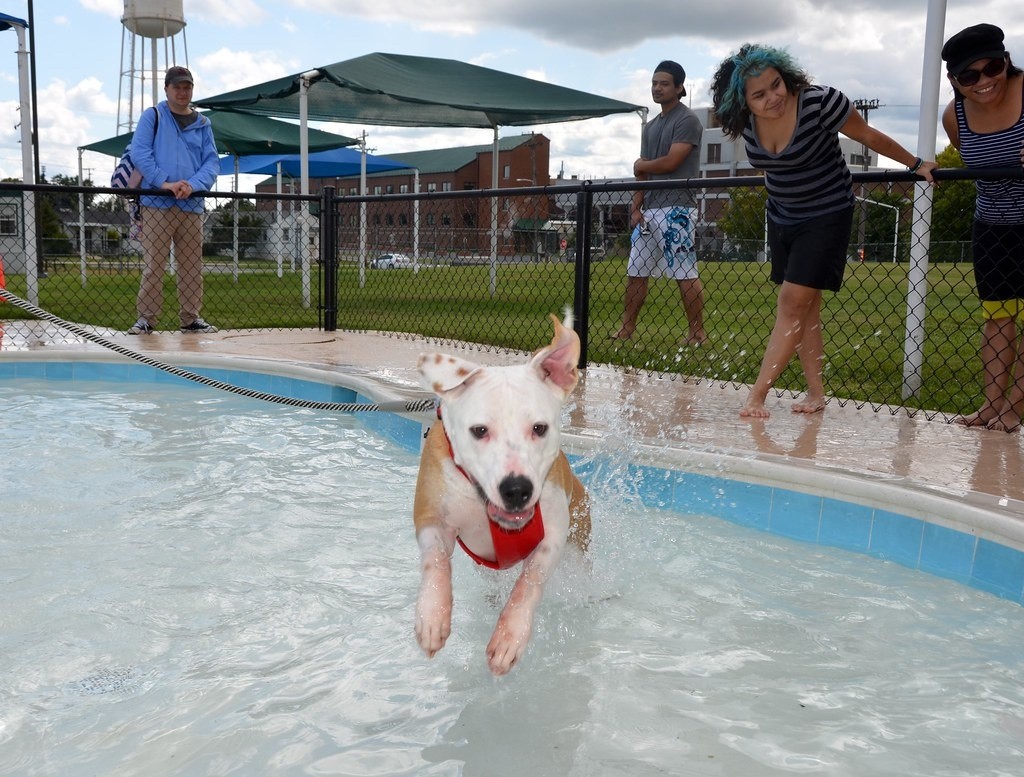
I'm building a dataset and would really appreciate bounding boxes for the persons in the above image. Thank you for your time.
[559,240,567,257]
[126,65,219,334]
[706,42,938,420]
[941,23,1024,433]
[609,60,711,346]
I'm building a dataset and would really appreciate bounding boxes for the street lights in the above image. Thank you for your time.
[515,178,538,262]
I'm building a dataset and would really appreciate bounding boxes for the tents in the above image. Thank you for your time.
[74,107,423,288]
[186,50,652,308]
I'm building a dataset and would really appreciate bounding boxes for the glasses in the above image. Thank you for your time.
[949,52,1006,87]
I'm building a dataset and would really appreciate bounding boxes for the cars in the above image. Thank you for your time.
[573,247,606,263]
[368,253,411,270]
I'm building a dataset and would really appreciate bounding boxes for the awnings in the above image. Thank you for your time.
[514,217,547,229]
[67,220,110,230]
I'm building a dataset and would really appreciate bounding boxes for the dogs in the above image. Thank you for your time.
[405,313,592,673]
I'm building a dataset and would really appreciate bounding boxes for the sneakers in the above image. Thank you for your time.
[180,320,218,334]
[128,320,154,335]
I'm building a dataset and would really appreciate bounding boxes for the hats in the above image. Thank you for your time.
[165,66,194,86]
[941,23,1006,72]
[654,60,687,97]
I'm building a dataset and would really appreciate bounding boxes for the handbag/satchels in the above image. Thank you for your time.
[111,107,159,196]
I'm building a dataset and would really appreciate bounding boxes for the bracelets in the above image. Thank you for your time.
[909,156,923,174]
